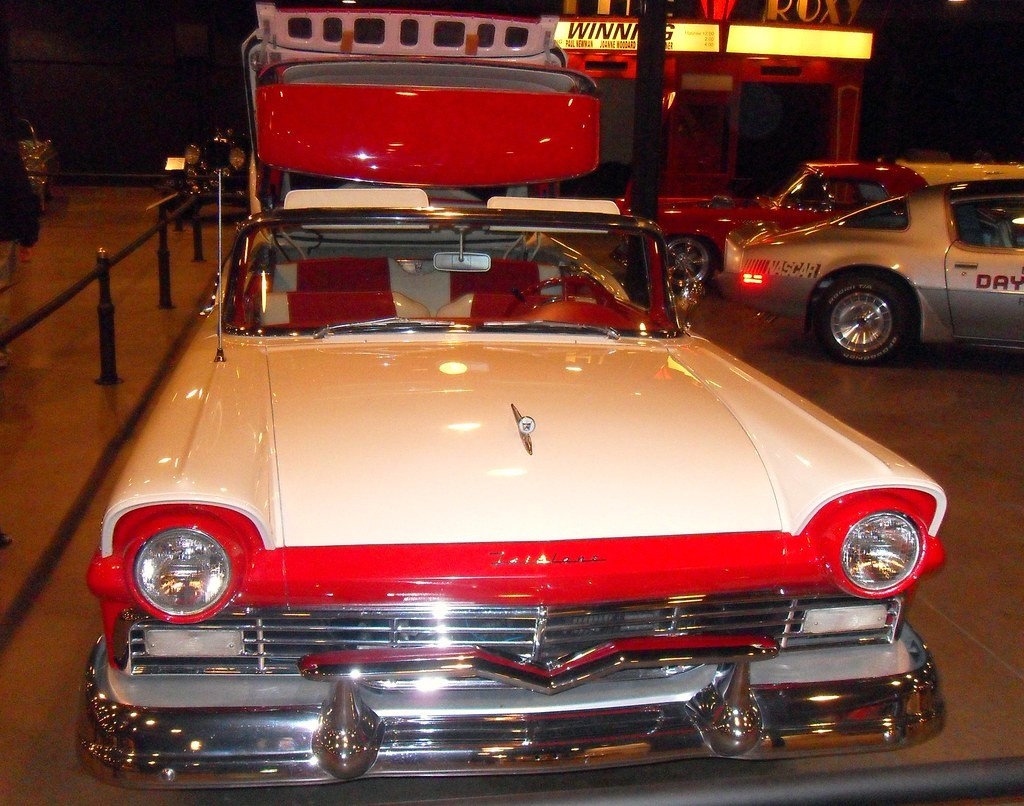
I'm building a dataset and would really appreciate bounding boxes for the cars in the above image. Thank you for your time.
[76,3,948,791]
[724,180,1024,366]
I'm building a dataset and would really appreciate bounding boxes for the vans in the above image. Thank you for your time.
[615,162,1024,294]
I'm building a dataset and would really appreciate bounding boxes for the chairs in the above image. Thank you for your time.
[436,293,598,318]
[260,291,430,326]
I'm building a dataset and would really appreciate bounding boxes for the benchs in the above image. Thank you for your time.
[997,220,1023,247]
[271,258,563,318]
